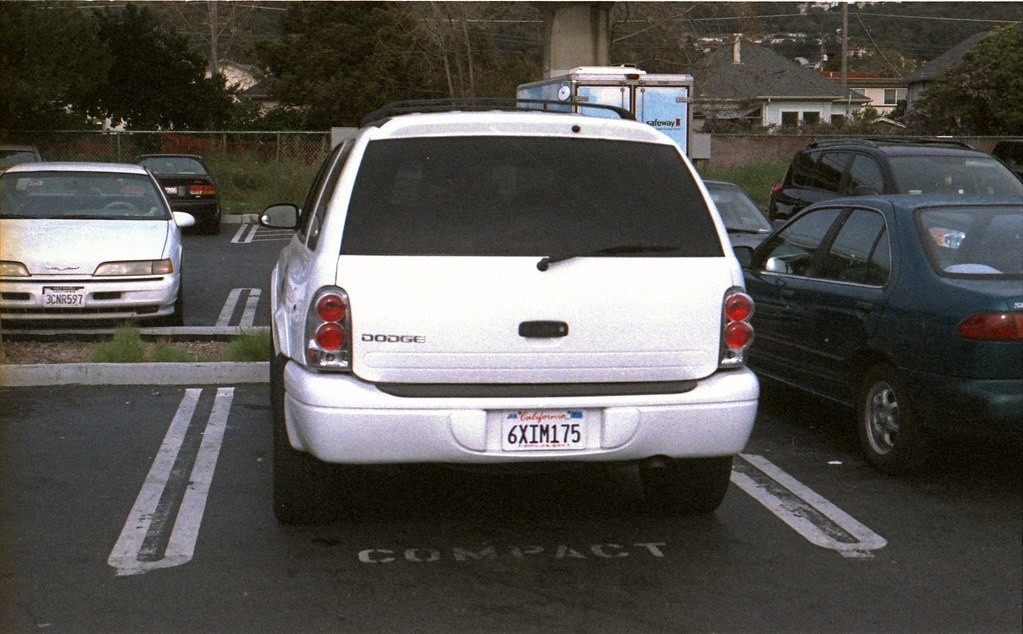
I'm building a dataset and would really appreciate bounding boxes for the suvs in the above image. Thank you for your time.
[260,93,762,524]
[769,137,1023,269]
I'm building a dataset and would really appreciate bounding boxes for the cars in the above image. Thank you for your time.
[0,161,196,328]
[702,178,788,250]
[0,143,54,197]
[118,153,223,235]
[730,191,1023,477]
